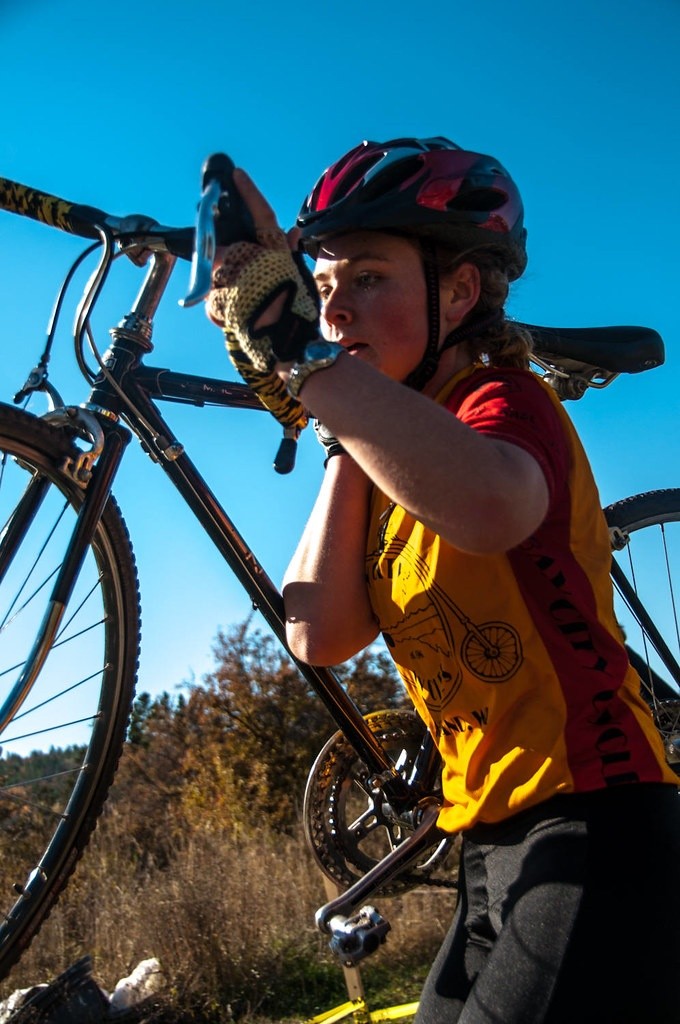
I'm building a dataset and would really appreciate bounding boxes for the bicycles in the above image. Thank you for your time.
[1,175,680,1024]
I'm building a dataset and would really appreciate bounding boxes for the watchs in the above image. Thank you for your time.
[287,339,348,398]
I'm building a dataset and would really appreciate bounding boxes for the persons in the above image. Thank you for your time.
[206,134,680,1024]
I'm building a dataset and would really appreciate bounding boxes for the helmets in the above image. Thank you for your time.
[294,136,527,273]
